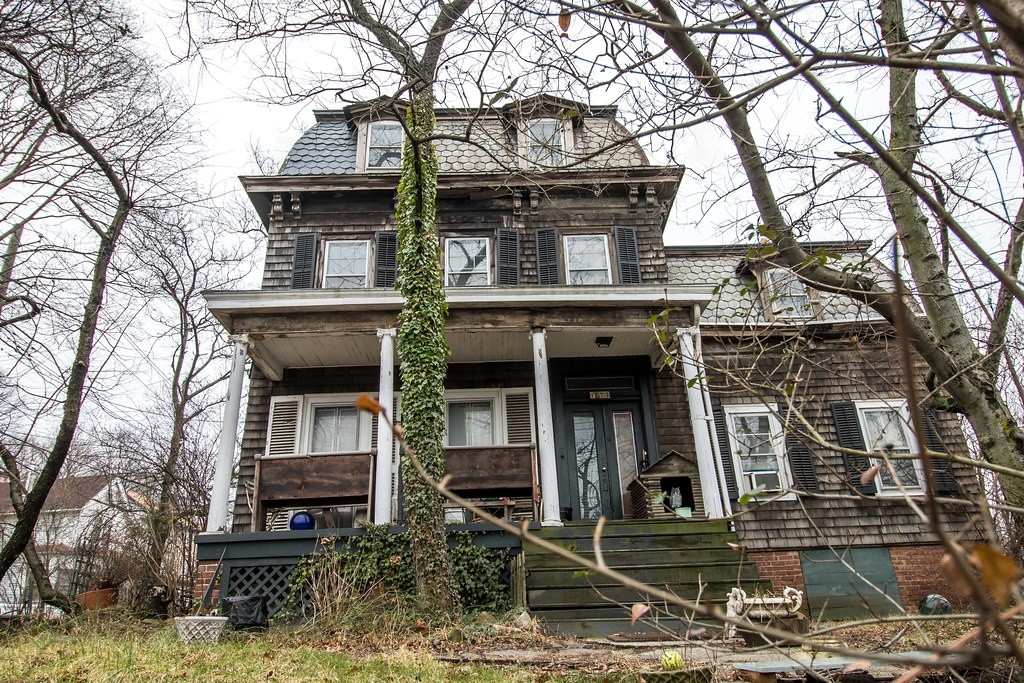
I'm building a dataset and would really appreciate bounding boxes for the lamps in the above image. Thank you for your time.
[595,337,614,347]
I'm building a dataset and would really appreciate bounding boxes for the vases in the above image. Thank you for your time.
[75,588,119,620]
